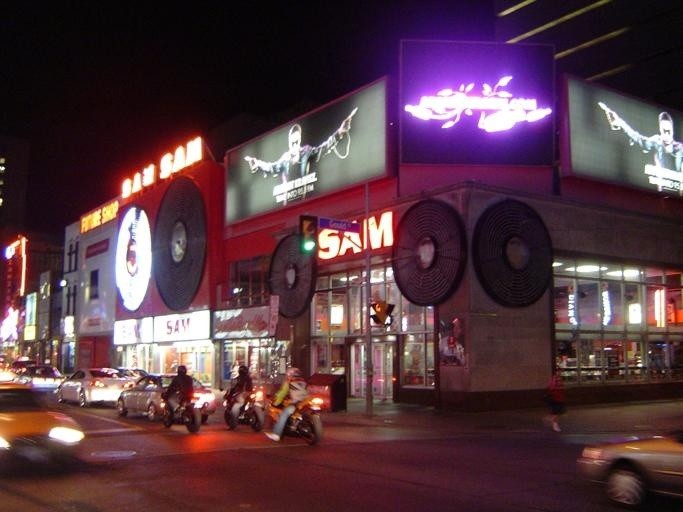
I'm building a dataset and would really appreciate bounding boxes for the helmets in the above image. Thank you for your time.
[176,365,187,377]
[284,366,303,382]
[237,365,249,376]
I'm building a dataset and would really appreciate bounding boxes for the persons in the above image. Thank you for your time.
[226,364,253,432]
[161,365,194,416]
[243,105,358,205]
[439,316,465,366]
[544,368,567,433]
[263,367,308,441]
[606,107,683,194]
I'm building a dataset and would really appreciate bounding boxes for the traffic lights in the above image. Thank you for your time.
[301,214,318,256]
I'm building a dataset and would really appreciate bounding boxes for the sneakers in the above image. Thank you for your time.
[263,431,280,443]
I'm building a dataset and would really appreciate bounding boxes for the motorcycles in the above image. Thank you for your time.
[223,390,264,433]
[265,397,322,444]
[160,393,201,432]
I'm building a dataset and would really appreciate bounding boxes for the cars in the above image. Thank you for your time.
[116,373,217,424]
[56,368,134,408]
[17,364,62,392]
[116,367,149,380]
[577,430,683,508]
